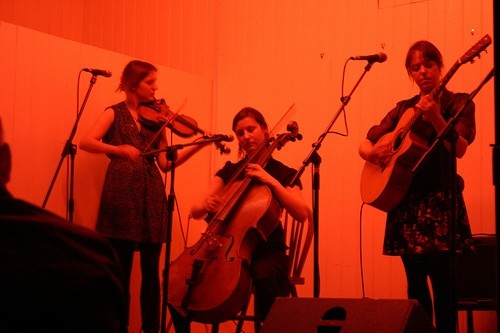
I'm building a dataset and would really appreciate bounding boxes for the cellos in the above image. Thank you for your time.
[165,120,303,322]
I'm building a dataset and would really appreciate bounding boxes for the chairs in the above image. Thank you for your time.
[210,203,313,333]
[458,233,500,333]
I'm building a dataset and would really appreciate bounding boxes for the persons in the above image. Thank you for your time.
[165,105,310,333]
[358,39,478,333]
[0,122,11,200]
[80,59,217,333]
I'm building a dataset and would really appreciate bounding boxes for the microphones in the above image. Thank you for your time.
[351,53,387,63]
[83,68,112,78]
[213,134,234,141]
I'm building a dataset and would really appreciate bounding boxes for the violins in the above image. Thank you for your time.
[139,99,232,153]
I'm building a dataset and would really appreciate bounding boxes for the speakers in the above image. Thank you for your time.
[261,297,434,333]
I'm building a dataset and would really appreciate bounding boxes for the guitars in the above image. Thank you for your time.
[359,34,492,211]
